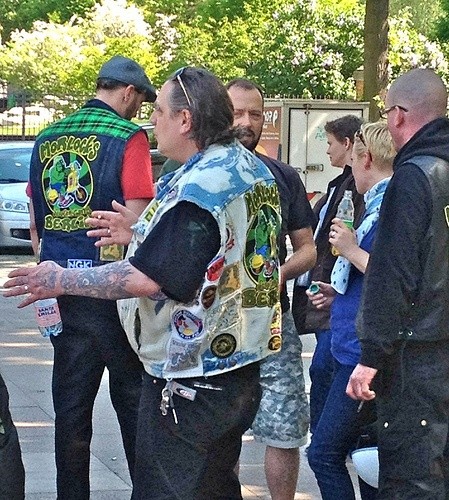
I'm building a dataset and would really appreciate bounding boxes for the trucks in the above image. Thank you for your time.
[256,97,370,212]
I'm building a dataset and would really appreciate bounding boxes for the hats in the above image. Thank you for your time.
[97,56,157,103]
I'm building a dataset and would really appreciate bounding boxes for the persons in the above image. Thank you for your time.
[225,80,317,500]
[0,374,25,500]
[291,70,449,500]
[28,55,159,500]
[4,67,283,500]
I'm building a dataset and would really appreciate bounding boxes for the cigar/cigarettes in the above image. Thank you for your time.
[98,215,102,219]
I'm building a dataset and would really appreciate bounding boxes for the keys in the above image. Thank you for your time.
[170,396,178,424]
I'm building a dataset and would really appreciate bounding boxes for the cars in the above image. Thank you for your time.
[0,140,37,249]
[139,123,169,164]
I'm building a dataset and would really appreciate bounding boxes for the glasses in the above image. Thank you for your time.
[172,67,191,107]
[356,129,372,162]
[379,105,408,118]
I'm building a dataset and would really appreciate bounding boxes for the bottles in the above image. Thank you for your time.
[26,262,64,337]
[331,190,355,257]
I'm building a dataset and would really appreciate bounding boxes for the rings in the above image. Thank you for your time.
[108,229,111,236]
[25,285,29,293]
